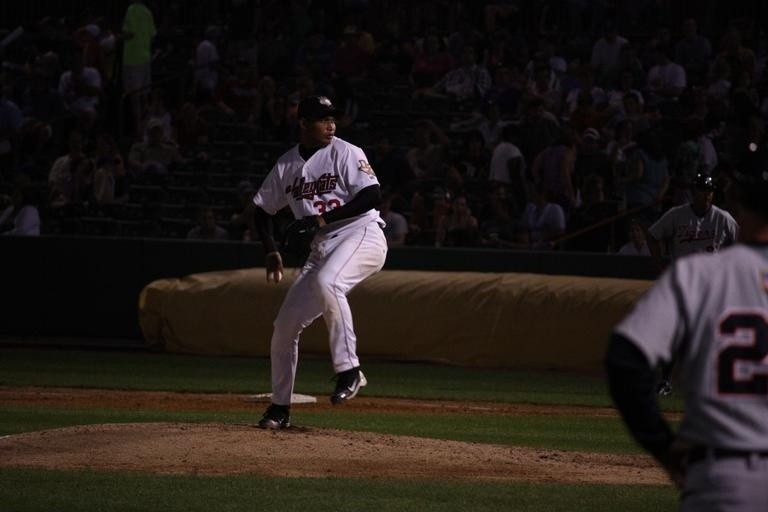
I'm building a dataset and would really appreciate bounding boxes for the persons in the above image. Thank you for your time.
[0,0,767,264]
[602,146,767,510]
[251,95,393,431]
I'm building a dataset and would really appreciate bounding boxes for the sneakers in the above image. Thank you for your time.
[655,379,672,396]
[327,367,368,404]
[259,403,290,430]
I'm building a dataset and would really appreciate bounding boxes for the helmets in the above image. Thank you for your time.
[692,173,718,191]
[725,134,768,188]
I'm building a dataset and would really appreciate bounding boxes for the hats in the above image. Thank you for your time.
[298,94,345,120]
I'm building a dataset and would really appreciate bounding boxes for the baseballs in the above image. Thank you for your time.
[270,271,283,282]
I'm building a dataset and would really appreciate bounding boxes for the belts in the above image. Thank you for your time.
[687,443,766,463]
